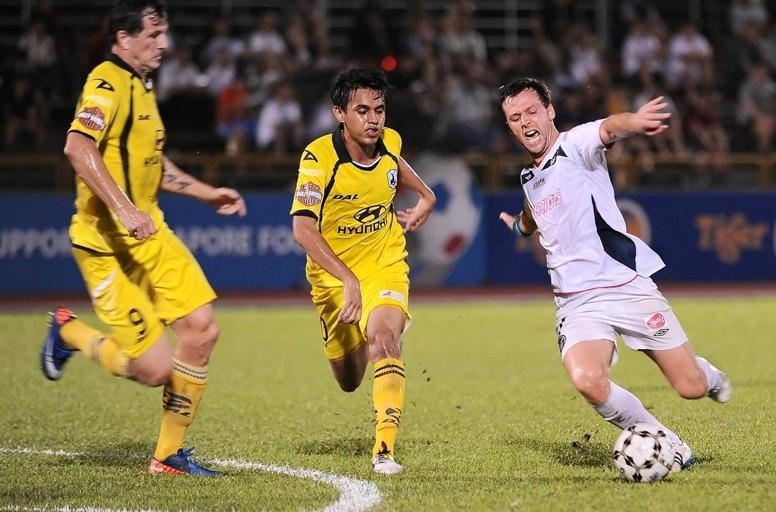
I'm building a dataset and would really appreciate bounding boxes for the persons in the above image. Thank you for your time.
[494,76,734,481]
[0,0,775,190]
[288,64,438,476]
[287,64,438,476]
[33,1,248,483]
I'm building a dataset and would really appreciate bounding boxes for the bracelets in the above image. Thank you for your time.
[513,219,532,240]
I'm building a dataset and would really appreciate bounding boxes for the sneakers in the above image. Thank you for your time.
[666,438,692,473]
[699,356,732,404]
[147,453,226,481]
[371,454,404,477]
[40,303,78,380]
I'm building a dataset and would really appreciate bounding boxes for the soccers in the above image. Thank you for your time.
[614,423,674,483]
